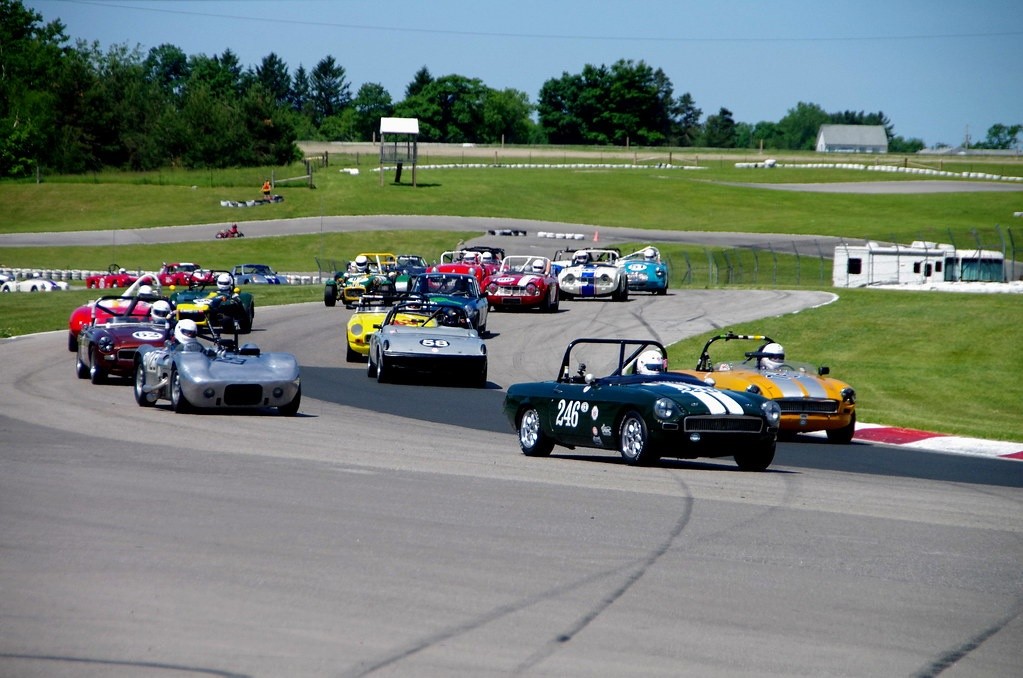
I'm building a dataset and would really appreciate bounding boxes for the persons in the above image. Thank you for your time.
[119,268,126,274]
[577,251,588,263]
[174,319,197,344]
[261,180,270,198]
[637,350,663,375]
[231,223,237,232]
[482,252,492,261]
[217,274,231,290]
[762,343,785,370]
[355,255,367,272]
[644,249,655,260]
[137,285,153,297]
[151,300,171,323]
[443,309,457,325]
[465,253,475,263]
[533,260,545,272]
[406,295,421,310]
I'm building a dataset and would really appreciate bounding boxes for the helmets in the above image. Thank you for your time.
[577,251,588,263]
[351,262,356,270]
[174,319,197,344]
[533,259,545,273]
[217,273,231,290]
[355,256,367,272]
[761,343,785,370]
[232,223,237,228]
[482,252,492,262]
[118,267,125,274]
[637,350,664,375]
[464,253,476,262]
[443,308,460,324]
[151,299,170,321]
[406,295,422,310]
[644,249,656,261]
[33,272,39,278]
[137,285,153,298]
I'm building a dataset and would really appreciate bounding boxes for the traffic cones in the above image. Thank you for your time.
[593,230,599,242]
[500,338,782,472]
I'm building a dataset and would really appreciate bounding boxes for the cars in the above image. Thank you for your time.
[230,264,290,286]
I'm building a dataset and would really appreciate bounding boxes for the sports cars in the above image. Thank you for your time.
[132,314,302,417]
[324,246,669,314]
[68,294,176,384]
[143,262,218,286]
[346,290,439,362]
[86,263,152,288]
[367,302,488,389]
[167,269,255,334]
[68,273,164,352]
[403,272,489,337]
[666,334,856,444]
[0,273,70,293]
